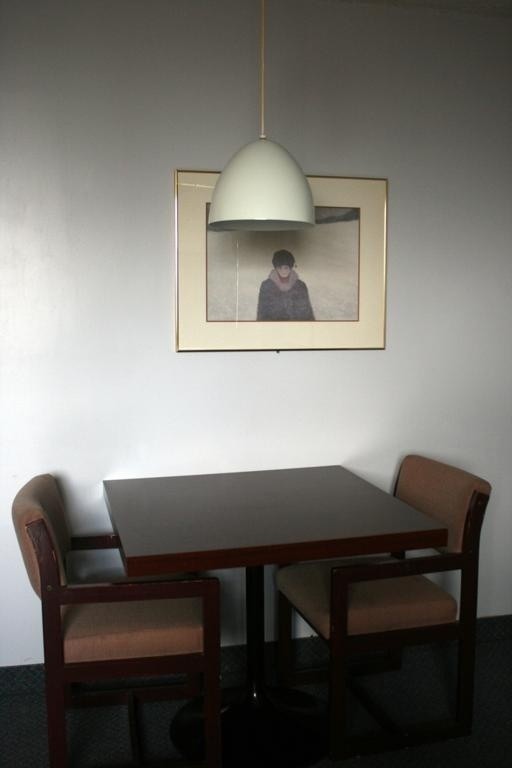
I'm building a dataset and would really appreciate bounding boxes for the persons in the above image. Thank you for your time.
[257,249,315,321]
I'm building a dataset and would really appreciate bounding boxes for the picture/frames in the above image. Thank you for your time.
[173,167,389,353]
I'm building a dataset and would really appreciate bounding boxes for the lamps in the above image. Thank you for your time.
[210,1,316,229]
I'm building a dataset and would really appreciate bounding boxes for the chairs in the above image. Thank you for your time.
[274,454,492,767]
[11,473,222,768]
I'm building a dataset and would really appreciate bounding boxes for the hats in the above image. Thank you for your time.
[272,249,295,268]
